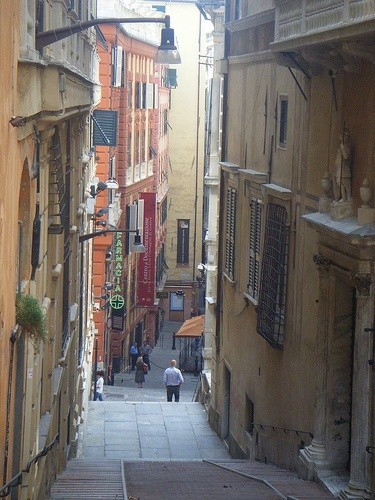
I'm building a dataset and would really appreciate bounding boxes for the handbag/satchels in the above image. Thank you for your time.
[143,365,147,374]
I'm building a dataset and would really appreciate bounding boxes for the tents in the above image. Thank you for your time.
[175,314,205,337]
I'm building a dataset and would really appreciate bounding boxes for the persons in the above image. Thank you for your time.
[139,341,152,370]
[92,371,104,401]
[130,342,138,371]
[135,357,147,388]
[163,360,184,402]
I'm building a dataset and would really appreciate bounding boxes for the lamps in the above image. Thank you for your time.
[35,0,182,64]
[79,228,146,254]
